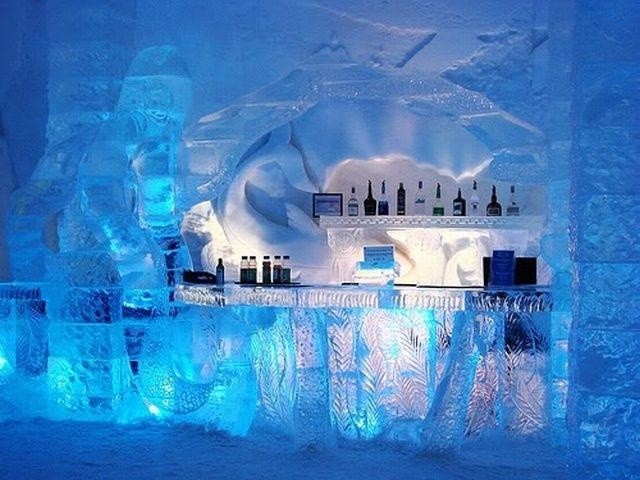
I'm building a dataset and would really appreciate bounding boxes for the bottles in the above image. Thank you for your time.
[378,179,389,215]
[240,256,291,284]
[216,258,224,284]
[348,188,359,217]
[363,180,377,216]
[486,185,502,216]
[468,180,481,216]
[414,180,425,216]
[396,182,406,215]
[432,183,444,216]
[452,187,466,216]
[506,185,520,217]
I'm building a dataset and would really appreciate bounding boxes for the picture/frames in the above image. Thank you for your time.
[312,193,343,218]
